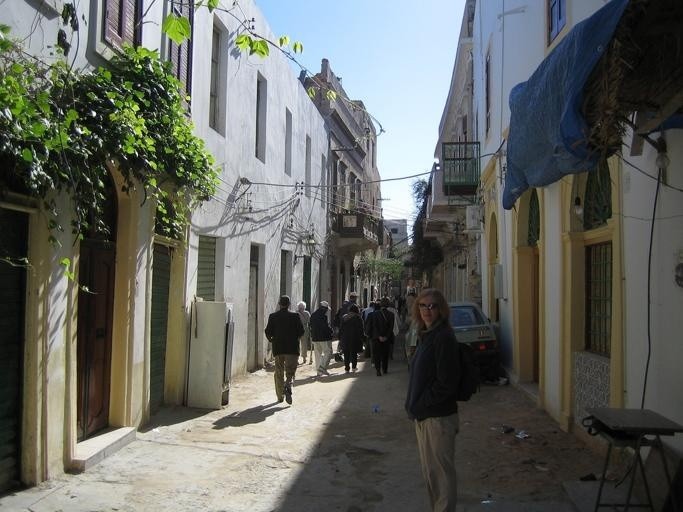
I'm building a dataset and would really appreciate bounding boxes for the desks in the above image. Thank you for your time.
[582,404,682,512]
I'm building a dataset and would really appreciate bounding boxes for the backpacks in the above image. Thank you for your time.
[455,340,480,403]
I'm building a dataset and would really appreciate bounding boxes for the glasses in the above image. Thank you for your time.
[417,302,441,310]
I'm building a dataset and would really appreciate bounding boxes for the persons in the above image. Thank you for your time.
[265,295,305,404]
[406,289,477,512]
[296,292,402,377]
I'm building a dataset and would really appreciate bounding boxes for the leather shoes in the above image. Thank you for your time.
[283,386,292,405]
[278,398,284,403]
[317,366,330,375]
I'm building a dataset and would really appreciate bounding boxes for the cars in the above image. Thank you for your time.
[405,302,496,376]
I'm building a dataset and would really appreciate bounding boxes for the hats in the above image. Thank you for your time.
[320,300,332,311]
